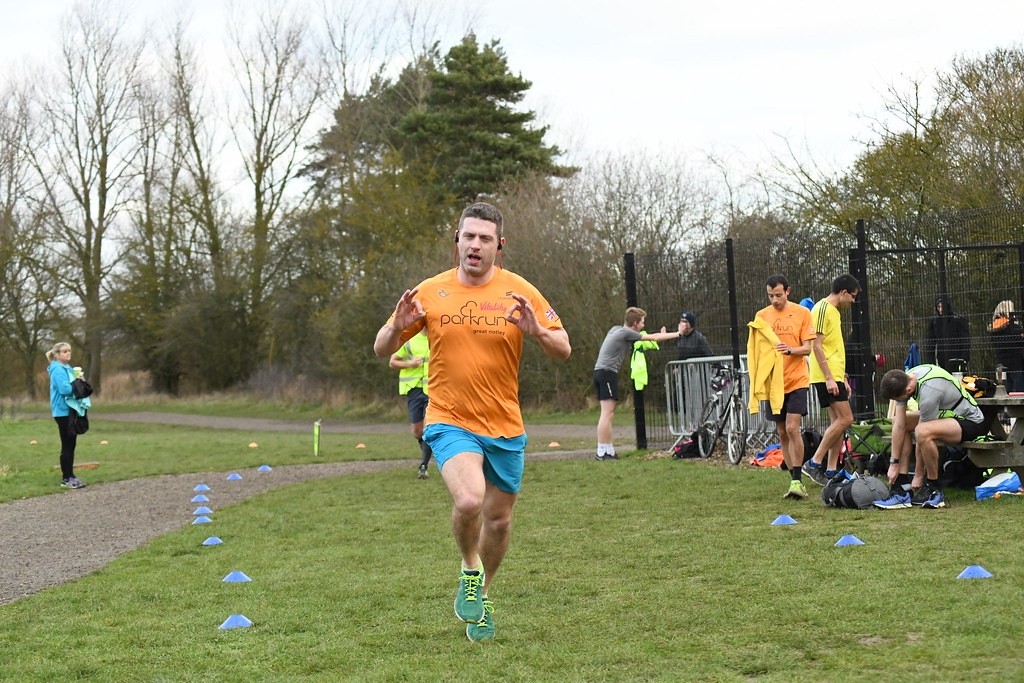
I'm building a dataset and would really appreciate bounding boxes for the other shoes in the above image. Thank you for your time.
[595,450,618,460]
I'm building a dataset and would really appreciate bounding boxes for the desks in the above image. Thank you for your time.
[974,396,1024,484]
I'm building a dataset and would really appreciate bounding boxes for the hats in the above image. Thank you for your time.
[681,313,695,327]
[799,297,814,312]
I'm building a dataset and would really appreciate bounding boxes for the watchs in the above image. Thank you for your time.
[890,458,899,463]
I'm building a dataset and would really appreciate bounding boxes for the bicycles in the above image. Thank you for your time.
[698,365,749,465]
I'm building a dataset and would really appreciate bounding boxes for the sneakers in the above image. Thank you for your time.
[874,489,913,510]
[912,478,942,504]
[824,471,839,480]
[801,460,830,486]
[417,438,423,450]
[61,476,86,489]
[454,554,486,624]
[922,490,946,509]
[418,463,429,480]
[784,480,809,498]
[465,592,497,642]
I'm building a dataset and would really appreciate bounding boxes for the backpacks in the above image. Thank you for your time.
[938,449,981,491]
[800,427,830,470]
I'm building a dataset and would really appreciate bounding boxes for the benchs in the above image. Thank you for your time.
[883,435,1013,469]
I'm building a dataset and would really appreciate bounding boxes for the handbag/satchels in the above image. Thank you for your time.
[975,472,1024,501]
[824,475,889,508]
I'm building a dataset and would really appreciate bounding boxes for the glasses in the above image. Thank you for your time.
[847,291,857,301]
[57,349,71,353]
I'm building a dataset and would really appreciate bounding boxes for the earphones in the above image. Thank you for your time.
[455,237,459,242]
[497,243,503,250]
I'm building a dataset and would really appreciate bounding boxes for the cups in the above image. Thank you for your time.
[73,366,82,379]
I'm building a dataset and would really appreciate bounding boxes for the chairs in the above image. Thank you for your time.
[845,397,918,459]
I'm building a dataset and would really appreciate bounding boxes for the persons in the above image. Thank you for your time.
[801,273,862,486]
[592,306,686,461]
[924,295,970,375]
[373,202,572,643]
[674,311,714,360]
[746,276,817,499]
[872,364,986,504]
[389,325,432,480]
[46,342,93,489]
[987,300,1024,395]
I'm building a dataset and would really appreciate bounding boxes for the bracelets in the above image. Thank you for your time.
[678,332,681,336]
[786,349,791,355]
